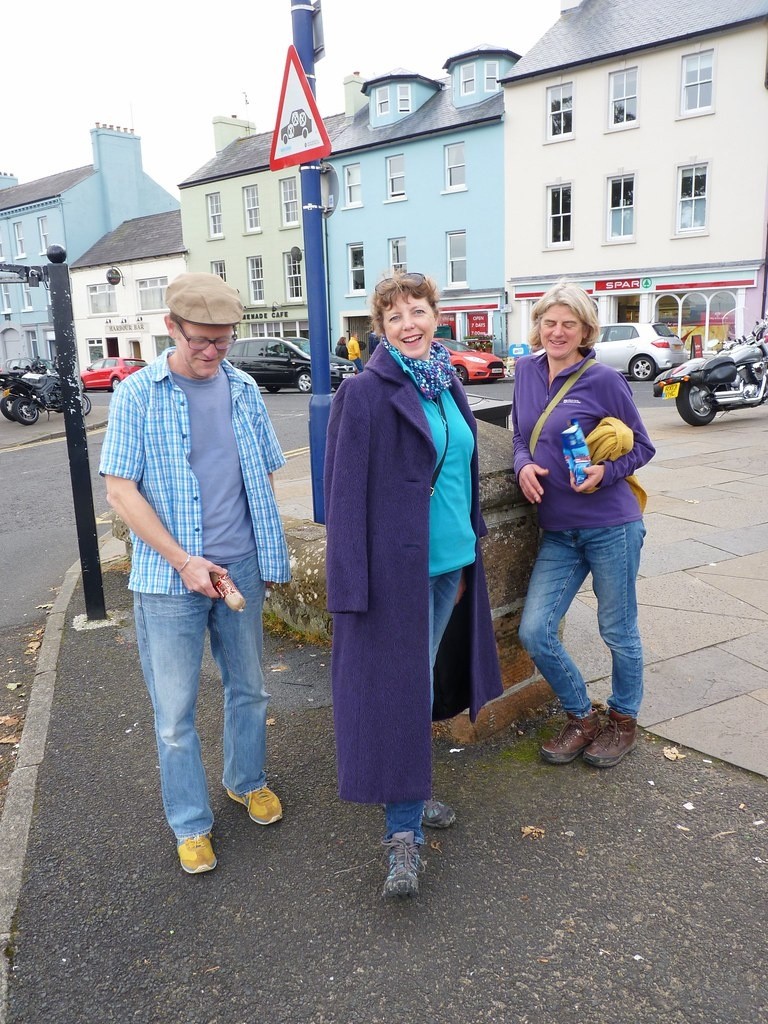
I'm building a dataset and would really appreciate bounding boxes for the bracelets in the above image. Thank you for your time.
[178,556,193,573]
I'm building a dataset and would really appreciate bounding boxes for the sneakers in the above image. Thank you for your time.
[583,709,638,767]
[540,708,602,763]
[177,833,217,874]
[227,789,283,825]
[382,803,456,827]
[381,831,426,897]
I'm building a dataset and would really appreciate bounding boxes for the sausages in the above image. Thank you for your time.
[210,572,246,612]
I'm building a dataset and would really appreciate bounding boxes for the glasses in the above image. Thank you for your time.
[376,273,427,295]
[175,320,238,351]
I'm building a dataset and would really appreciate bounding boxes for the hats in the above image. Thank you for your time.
[165,273,244,324]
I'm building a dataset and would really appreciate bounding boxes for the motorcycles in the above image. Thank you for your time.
[0,354,91,425]
[652,315,768,425]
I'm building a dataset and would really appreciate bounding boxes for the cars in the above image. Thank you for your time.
[431,337,505,384]
[80,357,149,392]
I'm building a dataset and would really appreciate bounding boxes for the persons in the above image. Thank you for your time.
[98,273,293,874]
[512,280,656,768]
[326,273,504,897]
[335,337,349,359]
[347,332,363,373]
[31,357,43,370]
[51,357,59,369]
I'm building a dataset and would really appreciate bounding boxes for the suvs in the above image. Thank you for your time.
[226,336,358,393]
[531,322,687,382]
